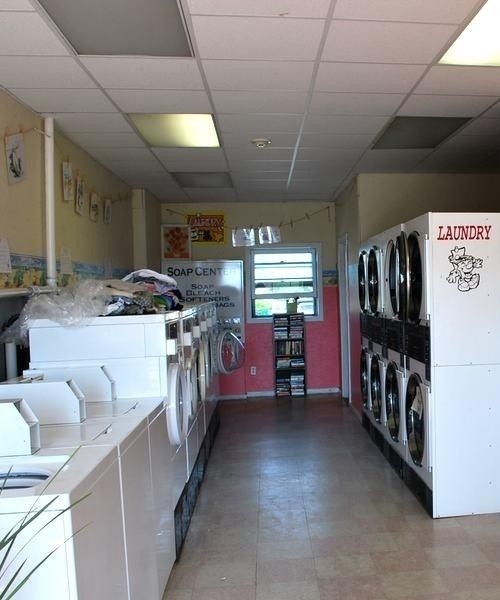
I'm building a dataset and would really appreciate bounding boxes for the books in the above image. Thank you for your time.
[273,315,305,396]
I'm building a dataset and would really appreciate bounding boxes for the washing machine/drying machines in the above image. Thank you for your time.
[0,301,245,600]
[356,211,500,519]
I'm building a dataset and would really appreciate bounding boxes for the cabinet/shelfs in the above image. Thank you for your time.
[272,312,306,399]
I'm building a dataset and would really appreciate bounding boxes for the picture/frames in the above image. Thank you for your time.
[161,224,192,261]
